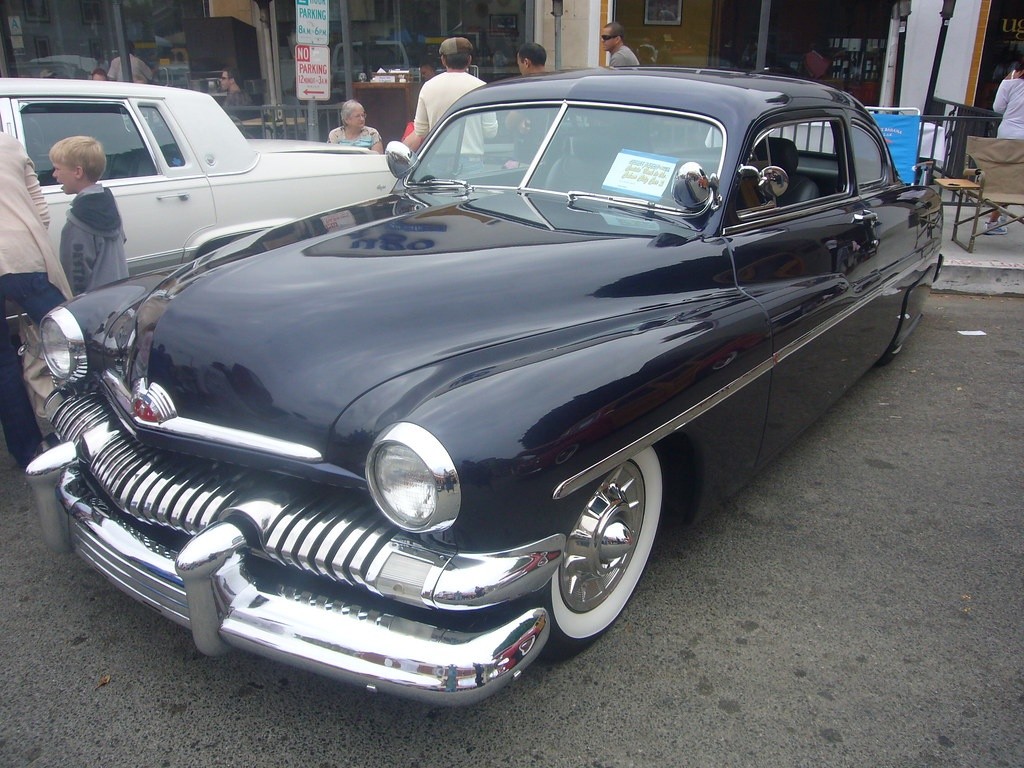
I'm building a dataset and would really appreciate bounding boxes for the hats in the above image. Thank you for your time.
[439,37,473,55]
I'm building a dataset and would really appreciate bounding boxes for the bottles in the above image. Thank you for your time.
[830,53,879,80]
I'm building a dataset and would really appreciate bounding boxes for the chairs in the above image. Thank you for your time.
[865,106,933,187]
[935,135,1024,253]
[753,137,818,206]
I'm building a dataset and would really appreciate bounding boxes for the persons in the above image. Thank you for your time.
[0,108,129,471]
[326,6,655,175]
[40,38,154,84]
[220,64,261,139]
[981,59,1024,235]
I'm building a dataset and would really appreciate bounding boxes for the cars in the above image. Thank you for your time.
[18,66,944,711]
[0,77,400,340]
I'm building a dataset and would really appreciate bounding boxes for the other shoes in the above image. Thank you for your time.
[983,222,1007,234]
[997,217,1008,225]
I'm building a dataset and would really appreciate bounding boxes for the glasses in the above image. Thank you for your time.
[602,35,618,41]
[353,114,367,120]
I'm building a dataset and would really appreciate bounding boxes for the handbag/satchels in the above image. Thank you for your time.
[15,314,57,438]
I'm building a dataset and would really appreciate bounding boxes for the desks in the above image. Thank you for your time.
[237,118,305,127]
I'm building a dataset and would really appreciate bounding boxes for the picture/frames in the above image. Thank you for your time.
[490,14,517,37]
[89,38,105,64]
[79,0,104,25]
[644,0,683,26]
[34,36,53,64]
[22,0,50,23]
